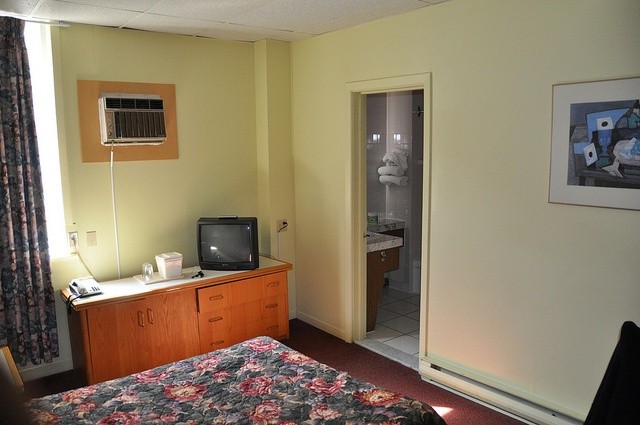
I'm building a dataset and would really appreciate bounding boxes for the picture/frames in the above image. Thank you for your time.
[548,77,640,210]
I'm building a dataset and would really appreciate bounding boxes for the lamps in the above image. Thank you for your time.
[367,132,381,150]
[392,132,409,148]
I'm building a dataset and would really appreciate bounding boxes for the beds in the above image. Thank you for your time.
[0,336,445,425]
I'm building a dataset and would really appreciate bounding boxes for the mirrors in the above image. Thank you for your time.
[366,91,387,216]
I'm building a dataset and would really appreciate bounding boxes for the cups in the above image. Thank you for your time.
[141,264,153,282]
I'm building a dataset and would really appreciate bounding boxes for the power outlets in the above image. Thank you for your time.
[276,218,288,231]
[68,230,80,251]
[86,231,97,247]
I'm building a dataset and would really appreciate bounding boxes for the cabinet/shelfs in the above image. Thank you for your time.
[196,252,294,354]
[60,268,200,387]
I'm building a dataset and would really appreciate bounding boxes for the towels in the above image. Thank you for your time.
[377,166,406,175]
[379,175,409,187]
[382,148,410,167]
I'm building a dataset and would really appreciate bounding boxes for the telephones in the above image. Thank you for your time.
[66,277,102,315]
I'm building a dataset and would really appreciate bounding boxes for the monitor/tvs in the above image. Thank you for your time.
[196,217,259,271]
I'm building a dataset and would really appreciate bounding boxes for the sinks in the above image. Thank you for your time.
[370,216,406,247]
